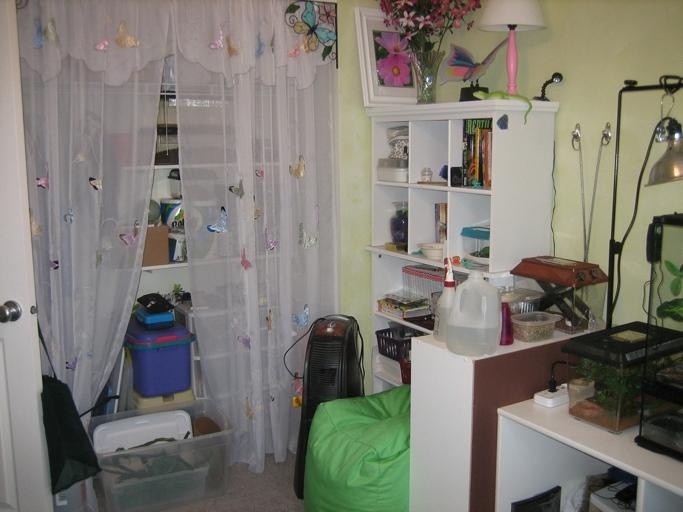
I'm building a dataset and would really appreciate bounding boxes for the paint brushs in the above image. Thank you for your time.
[534,376,597,408]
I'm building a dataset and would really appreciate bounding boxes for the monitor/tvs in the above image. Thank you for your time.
[377,291,431,320]
[461,117,494,190]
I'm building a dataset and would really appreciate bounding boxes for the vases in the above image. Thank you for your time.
[376,30,411,88]
[380,1,484,49]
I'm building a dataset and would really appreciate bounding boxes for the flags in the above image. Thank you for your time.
[407,50,448,103]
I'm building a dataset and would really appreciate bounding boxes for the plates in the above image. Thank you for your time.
[408,307,617,512]
[366,99,556,393]
[26,16,310,431]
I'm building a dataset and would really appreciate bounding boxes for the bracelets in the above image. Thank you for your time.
[420,243,443,260]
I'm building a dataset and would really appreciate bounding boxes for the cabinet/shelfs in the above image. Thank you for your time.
[421,167,433,183]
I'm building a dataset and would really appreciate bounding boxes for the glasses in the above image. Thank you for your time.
[478,0,547,95]
[604,74,683,329]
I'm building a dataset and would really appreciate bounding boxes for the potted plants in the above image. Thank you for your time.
[353,7,419,107]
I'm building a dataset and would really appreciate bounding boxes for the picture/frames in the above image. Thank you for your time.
[495,370,683,512]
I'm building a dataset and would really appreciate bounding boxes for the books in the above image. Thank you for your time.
[85,399,238,511]
[125,322,197,397]
